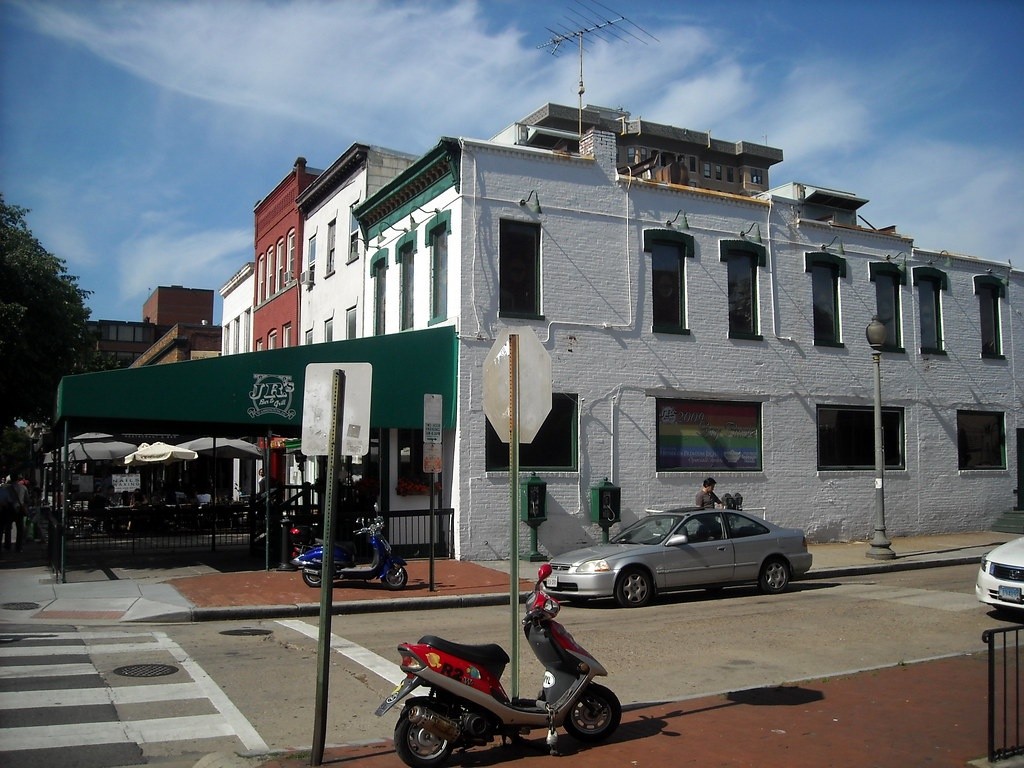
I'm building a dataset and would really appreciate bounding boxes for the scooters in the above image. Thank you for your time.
[290,501,409,591]
[372,564,623,768]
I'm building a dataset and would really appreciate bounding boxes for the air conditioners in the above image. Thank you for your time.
[284,271,294,284]
[300,271,314,285]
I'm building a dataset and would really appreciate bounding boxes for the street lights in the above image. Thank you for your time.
[864,315,898,560]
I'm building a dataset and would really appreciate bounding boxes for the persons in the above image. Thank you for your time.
[696,477,724,533]
[0,471,44,553]
[88,469,277,536]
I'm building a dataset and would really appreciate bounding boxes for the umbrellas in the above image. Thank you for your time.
[43,432,263,465]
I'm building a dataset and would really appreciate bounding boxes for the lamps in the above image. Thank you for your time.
[518,189,543,215]
[986,267,1009,286]
[739,222,763,244]
[351,238,380,257]
[408,205,440,230]
[666,208,690,230]
[376,221,408,242]
[885,251,907,272]
[822,236,845,255]
[926,250,953,268]
[172,336,189,344]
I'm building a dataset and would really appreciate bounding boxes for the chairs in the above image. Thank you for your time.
[674,524,689,538]
[66,505,253,536]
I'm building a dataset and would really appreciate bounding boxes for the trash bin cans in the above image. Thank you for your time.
[23,516,35,542]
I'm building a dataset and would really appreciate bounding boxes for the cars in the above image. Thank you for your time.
[975,538,1024,615]
[545,507,814,610]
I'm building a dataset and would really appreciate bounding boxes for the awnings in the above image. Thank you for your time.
[54,326,458,579]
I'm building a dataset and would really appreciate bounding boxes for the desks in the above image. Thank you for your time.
[105,505,134,532]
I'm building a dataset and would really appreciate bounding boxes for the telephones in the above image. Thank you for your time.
[530,487,539,505]
[602,491,610,509]
